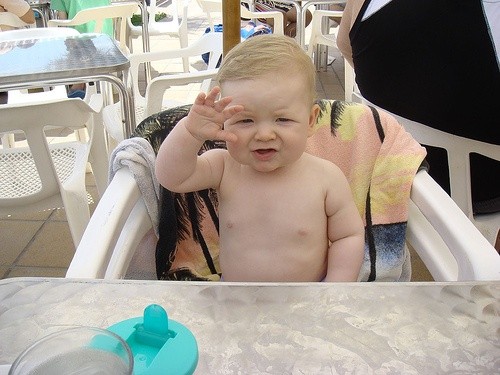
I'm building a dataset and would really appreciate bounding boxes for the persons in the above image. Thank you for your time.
[155,34,364,283]
[240,0,312,38]
[0,0,115,100]
[337,0,500,256]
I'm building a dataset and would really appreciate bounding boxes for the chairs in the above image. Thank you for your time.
[65,99,500,280]
[0,0,353,248]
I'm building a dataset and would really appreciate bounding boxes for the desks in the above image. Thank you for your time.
[0,33,136,138]
[0,275,500,375]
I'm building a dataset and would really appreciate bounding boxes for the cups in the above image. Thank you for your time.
[9,326,134,375]
